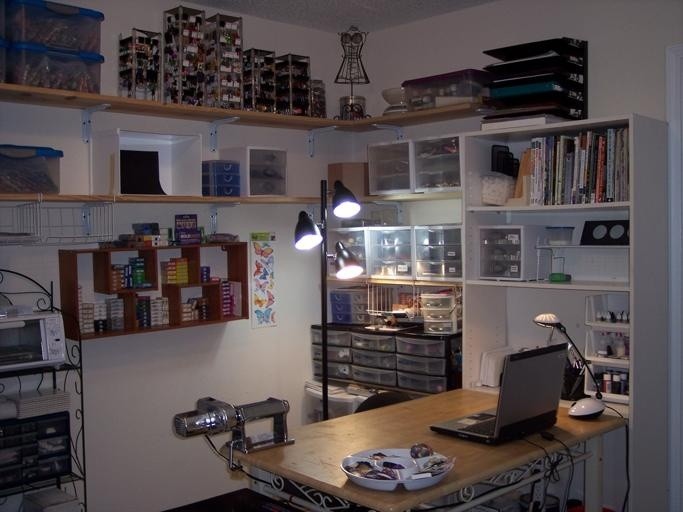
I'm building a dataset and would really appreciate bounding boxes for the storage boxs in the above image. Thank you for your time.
[0,1,105,56]
[0,143,66,194]
[0,43,103,93]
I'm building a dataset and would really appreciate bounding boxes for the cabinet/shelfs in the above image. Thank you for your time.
[462,112,672,393]
[584,288,628,400]
[111,4,329,118]
[0,81,320,219]
[59,242,250,341]
[0,267,87,509]
[314,133,463,392]
[480,225,553,283]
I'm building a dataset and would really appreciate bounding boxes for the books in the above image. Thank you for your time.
[513,127,629,206]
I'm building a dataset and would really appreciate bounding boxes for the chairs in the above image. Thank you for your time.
[355,385,411,413]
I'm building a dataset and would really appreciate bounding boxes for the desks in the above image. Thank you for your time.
[219,387,626,511]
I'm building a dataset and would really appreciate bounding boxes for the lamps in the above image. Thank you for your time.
[291,177,373,423]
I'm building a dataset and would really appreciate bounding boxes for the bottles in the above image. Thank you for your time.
[593,369,629,394]
[598,329,626,359]
[596,309,630,325]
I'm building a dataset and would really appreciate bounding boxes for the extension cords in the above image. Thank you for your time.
[532,459,545,512]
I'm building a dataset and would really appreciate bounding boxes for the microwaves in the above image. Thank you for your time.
[0,309,67,374]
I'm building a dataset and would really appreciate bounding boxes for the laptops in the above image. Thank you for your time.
[430,343,568,446]
